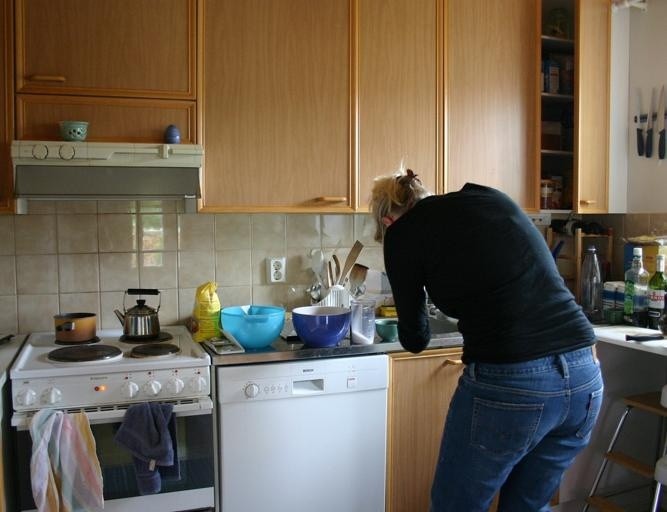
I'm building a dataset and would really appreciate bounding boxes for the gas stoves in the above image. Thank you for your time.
[9,325,211,410]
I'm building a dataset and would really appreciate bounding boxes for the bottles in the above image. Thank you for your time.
[623,248,650,327]
[580,243,604,325]
[648,255,667,331]
[164,124,181,144]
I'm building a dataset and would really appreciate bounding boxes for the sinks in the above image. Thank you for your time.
[372,314,460,344]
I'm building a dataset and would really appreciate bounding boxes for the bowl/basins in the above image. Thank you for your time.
[375,319,399,343]
[292,306,352,349]
[220,304,287,349]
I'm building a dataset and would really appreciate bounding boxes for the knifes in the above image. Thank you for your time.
[636,85,666,159]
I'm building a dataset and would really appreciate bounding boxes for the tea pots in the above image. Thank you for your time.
[113,288,161,342]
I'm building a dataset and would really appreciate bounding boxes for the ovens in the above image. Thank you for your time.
[11,398,215,512]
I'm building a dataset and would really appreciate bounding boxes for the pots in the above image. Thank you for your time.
[53,313,97,344]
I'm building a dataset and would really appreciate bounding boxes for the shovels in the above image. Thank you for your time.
[338,240,365,285]
[349,264,370,294]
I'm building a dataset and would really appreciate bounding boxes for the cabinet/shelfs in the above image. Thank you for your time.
[537,0,609,217]
[355,0,539,215]
[386,345,561,512]
[0,0,17,215]
[199,0,356,213]
[15,0,197,146]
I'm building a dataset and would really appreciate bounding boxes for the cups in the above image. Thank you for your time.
[350,299,376,346]
[606,308,624,325]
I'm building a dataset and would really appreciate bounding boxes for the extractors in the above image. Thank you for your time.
[10,140,205,198]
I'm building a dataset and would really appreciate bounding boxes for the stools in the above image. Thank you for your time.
[581,397,667,512]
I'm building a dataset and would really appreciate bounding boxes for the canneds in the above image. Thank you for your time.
[541,179,553,209]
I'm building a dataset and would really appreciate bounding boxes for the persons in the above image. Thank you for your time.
[370,168,605,512]
